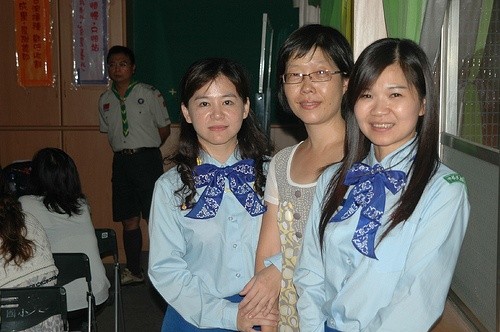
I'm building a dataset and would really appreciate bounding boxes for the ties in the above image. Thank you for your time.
[111,80,139,137]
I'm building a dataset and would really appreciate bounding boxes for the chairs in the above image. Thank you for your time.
[0,252,95,332]
[95,228,125,332]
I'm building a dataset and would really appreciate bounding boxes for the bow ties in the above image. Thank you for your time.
[329,162,407,261]
[184,158,267,219]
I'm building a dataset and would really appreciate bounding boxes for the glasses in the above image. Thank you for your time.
[281,71,341,84]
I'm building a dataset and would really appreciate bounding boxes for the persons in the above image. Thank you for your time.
[98,45,171,286]
[0,147,110,332]
[146,23,470,332]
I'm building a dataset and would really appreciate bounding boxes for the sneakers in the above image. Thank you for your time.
[121,269,144,285]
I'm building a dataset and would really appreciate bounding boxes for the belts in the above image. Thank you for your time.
[114,147,160,156]
